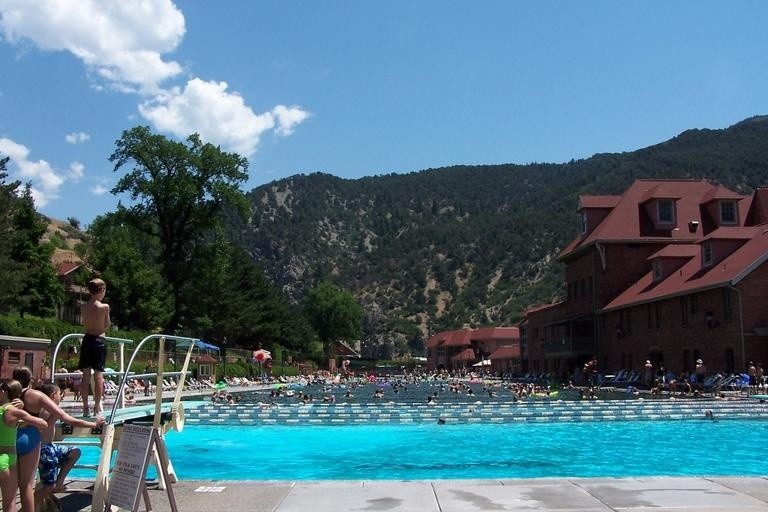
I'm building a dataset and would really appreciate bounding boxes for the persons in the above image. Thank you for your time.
[194,358,764,427]
[0,278,153,512]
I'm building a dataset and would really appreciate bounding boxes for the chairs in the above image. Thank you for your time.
[279,375,301,383]
[223,377,258,386]
[607,369,737,391]
[104,376,208,395]
